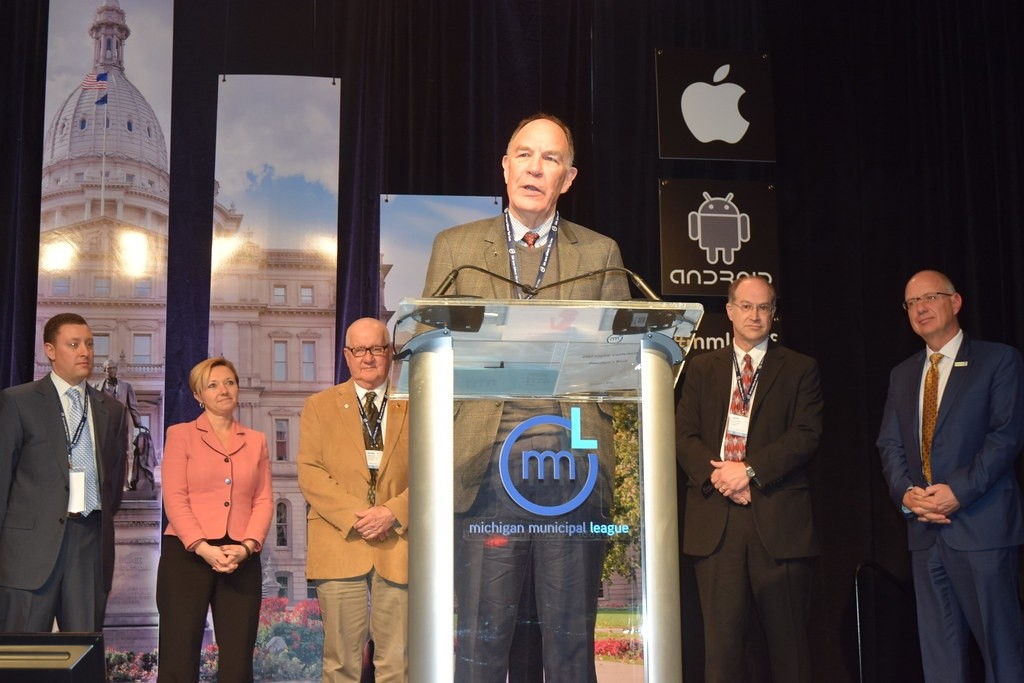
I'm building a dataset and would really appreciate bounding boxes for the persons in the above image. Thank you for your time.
[414,114,633,683]
[156,358,275,683]
[0,313,128,636]
[297,318,410,683]
[91,359,149,491]
[675,276,819,683]
[876,271,1024,683]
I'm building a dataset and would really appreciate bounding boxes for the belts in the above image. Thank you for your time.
[65,510,101,521]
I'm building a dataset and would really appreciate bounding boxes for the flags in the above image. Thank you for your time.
[94,93,108,106]
[80,73,107,89]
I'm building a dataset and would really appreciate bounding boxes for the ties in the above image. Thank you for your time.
[522,232,540,249]
[725,352,753,462]
[65,386,98,517]
[920,353,948,483]
[361,392,384,506]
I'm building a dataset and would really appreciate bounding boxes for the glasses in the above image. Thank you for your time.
[346,344,389,357]
[734,302,772,316]
[903,291,952,311]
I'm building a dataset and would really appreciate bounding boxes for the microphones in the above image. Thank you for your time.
[411,264,675,335]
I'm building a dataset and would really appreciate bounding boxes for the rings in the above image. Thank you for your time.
[368,530,370,535]
[722,483,728,492]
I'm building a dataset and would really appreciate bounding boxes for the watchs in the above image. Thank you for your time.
[742,459,755,478]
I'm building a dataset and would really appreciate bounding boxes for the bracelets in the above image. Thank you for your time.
[240,543,251,558]
